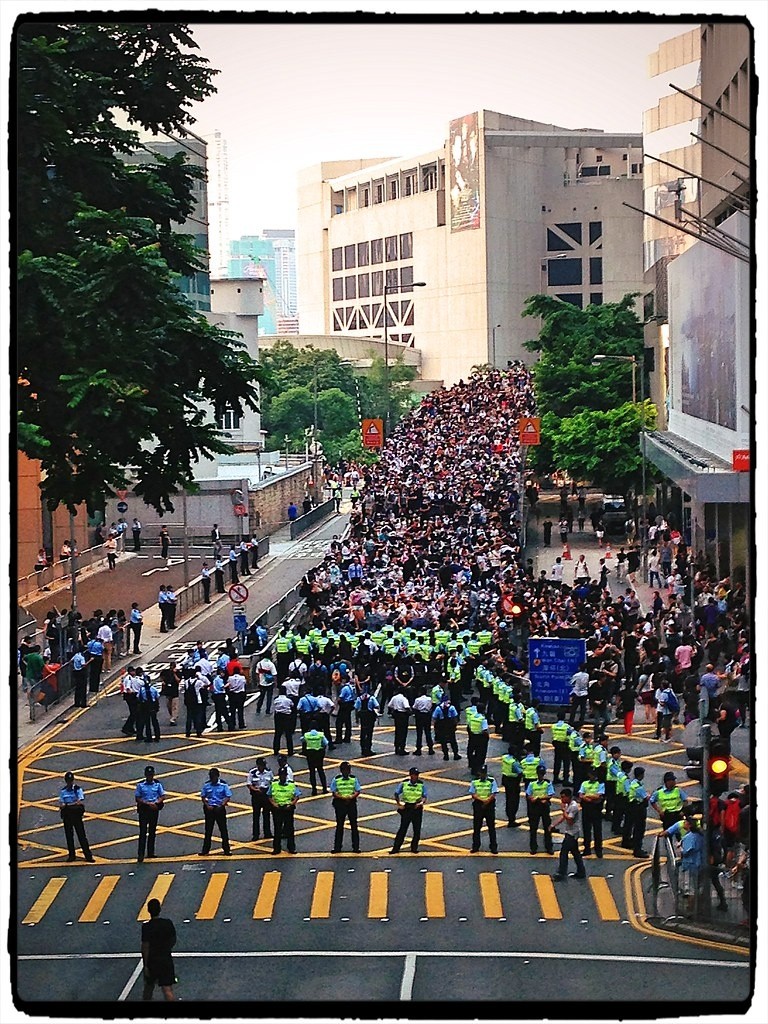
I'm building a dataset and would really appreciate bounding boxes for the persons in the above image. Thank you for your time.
[546,787,588,880]
[331,762,363,854]
[301,721,332,796]
[245,756,301,855]
[136,766,166,862]
[197,767,235,857]
[142,898,178,1002]
[578,764,607,859]
[525,766,556,855]
[122,364,756,755]
[468,764,503,855]
[550,708,754,928]
[465,695,490,774]
[389,767,426,855]
[499,743,546,827]
[58,773,95,863]
[18,517,261,708]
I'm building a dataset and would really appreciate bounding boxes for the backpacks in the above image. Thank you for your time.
[184,679,198,705]
[289,660,304,679]
[661,691,678,712]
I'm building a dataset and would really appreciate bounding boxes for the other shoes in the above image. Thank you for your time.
[739,918,749,926]
[122,725,462,760]
[63,818,653,863]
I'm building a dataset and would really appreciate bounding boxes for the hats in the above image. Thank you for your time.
[202,562,209,566]
[167,584,173,590]
[478,764,488,771]
[277,767,288,776]
[208,768,219,775]
[144,766,154,776]
[65,772,75,781]
[536,764,546,771]
[664,772,677,783]
[339,761,351,769]
[410,767,423,775]
[501,672,512,680]
[255,757,268,765]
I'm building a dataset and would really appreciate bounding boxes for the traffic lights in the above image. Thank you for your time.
[709,737,730,798]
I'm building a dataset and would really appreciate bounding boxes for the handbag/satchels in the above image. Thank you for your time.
[259,663,275,684]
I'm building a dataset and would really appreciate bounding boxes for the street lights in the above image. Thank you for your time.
[493,323,501,365]
[383,280,426,369]
[594,355,649,583]
